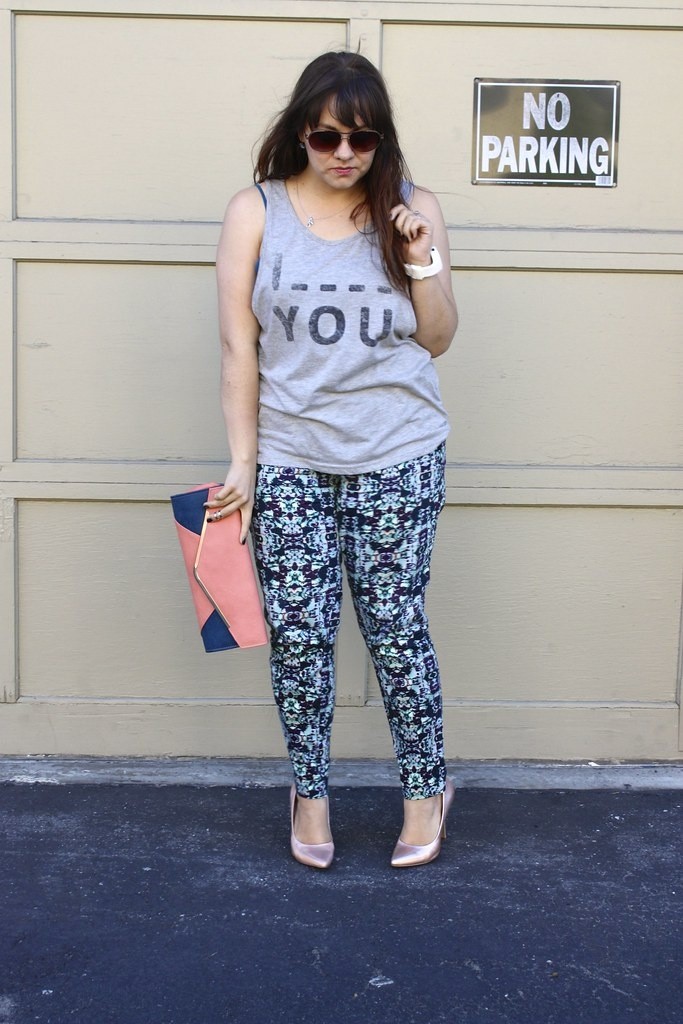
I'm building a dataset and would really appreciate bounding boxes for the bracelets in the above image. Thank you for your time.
[401,247,443,280]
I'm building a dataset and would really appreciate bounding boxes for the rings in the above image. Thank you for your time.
[414,210,421,219]
[214,512,220,520]
[218,510,224,519]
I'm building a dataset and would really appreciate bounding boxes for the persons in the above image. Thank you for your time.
[212,51,463,875]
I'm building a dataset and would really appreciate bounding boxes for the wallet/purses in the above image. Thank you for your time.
[169,481,269,654]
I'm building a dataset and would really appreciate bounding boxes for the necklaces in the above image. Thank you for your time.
[295,174,362,228]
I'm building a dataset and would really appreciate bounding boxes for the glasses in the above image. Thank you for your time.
[301,122,385,154]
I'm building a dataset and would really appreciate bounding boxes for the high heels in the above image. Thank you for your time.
[389,774,456,868]
[286,780,336,871]
